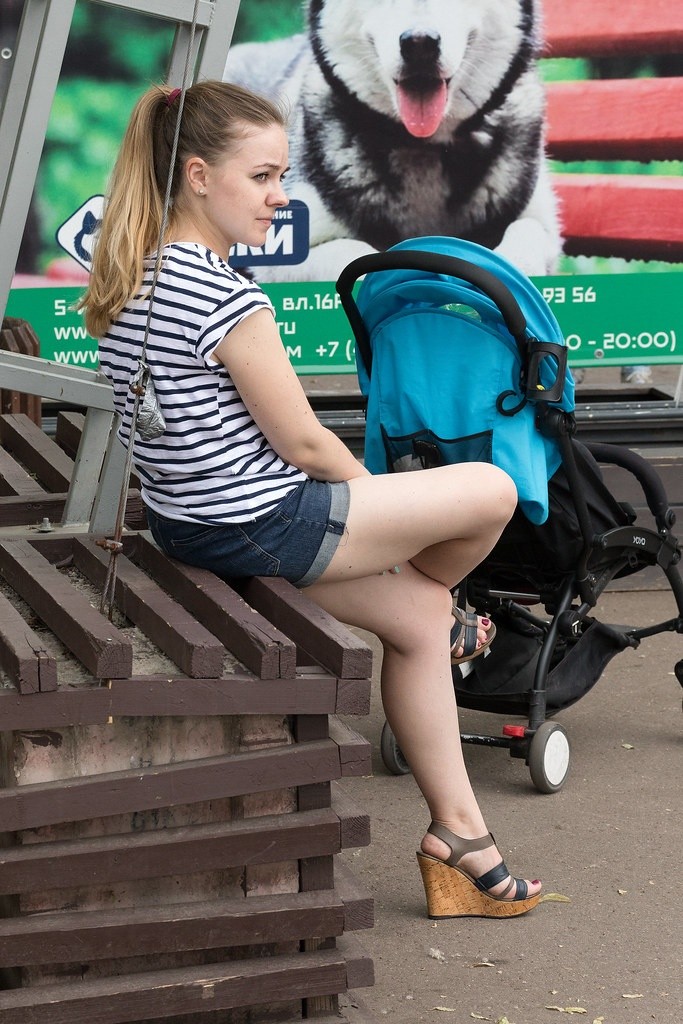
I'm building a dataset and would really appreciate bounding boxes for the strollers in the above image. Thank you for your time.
[334,236,683,795]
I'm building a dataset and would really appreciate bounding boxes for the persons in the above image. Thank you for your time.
[78,82,544,918]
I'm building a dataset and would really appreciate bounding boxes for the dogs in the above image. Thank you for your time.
[220,0,564,279]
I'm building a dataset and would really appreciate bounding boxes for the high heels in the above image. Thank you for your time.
[416,820,544,920]
[449,604,497,666]
